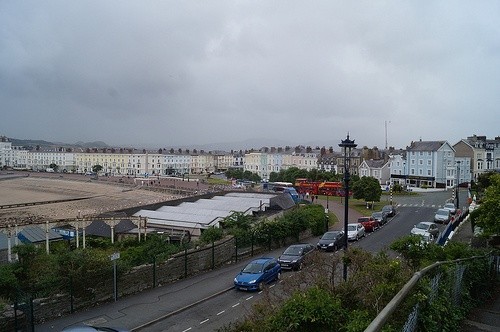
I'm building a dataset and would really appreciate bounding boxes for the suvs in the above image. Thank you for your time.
[371,211,387,226]
[380,205,396,217]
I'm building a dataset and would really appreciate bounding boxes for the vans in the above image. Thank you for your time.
[356,216,380,232]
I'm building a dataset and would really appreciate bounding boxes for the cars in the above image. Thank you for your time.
[341,223,365,241]
[443,203,456,216]
[233,257,282,293]
[277,242,318,272]
[317,230,348,253]
[406,231,434,250]
[409,221,439,239]
[433,208,451,224]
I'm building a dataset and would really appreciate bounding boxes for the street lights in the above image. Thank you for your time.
[338,133,358,284]
